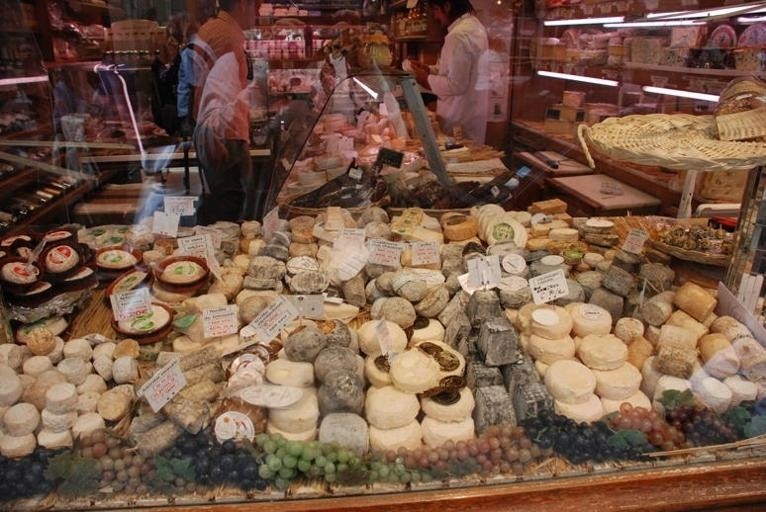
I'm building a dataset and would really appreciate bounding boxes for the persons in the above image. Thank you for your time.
[189,2,266,224]
[174,23,220,141]
[474,35,506,96]
[402,0,491,156]
[153,31,186,136]
[48,81,85,171]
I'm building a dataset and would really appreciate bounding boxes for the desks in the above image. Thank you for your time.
[1,216,764,509]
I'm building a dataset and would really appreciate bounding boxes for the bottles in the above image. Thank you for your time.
[392,7,428,37]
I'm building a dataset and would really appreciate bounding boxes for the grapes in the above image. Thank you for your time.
[0,397,766,501]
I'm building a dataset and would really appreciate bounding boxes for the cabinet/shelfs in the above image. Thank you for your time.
[3,1,766,237]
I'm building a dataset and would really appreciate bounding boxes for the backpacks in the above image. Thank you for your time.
[151,43,213,138]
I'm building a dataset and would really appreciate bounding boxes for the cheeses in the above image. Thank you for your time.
[713,97,766,138]
[0,198,766,458]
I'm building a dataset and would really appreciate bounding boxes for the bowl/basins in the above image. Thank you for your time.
[0,228,210,344]
